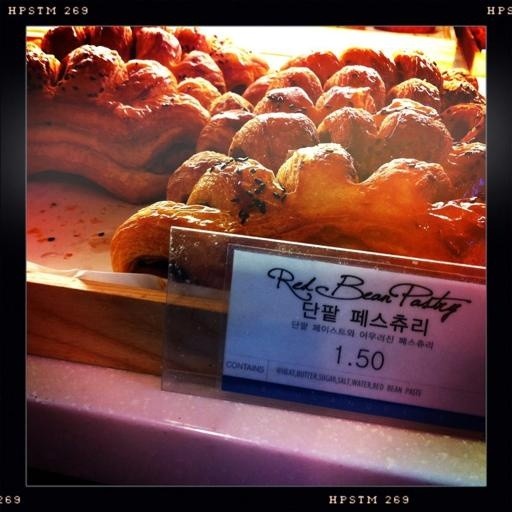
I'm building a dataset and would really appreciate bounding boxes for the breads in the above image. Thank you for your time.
[26,26,487,292]
